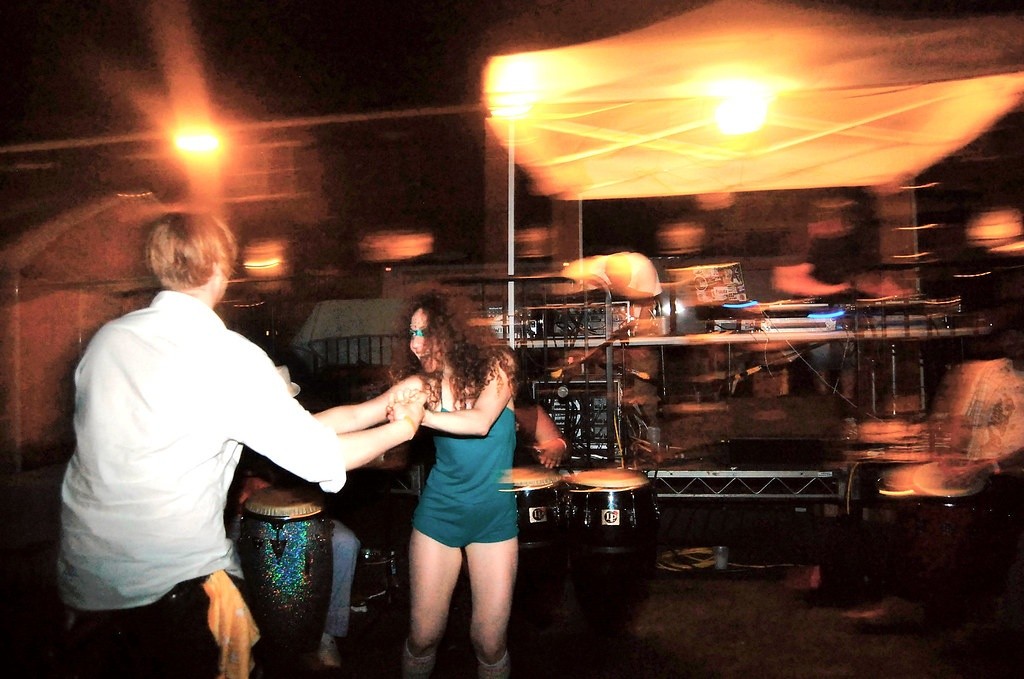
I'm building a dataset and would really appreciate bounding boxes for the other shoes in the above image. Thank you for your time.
[315,632,342,668]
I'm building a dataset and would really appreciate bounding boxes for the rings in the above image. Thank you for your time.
[537,448,541,454]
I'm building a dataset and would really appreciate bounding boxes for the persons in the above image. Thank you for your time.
[311,286,519,679]
[368,369,571,467]
[240,365,359,666]
[60,214,424,679]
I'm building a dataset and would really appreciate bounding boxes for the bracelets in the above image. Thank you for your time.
[559,438,567,450]
[404,417,416,434]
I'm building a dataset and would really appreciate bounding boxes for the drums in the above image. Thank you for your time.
[237,478,344,668]
[483,459,675,610]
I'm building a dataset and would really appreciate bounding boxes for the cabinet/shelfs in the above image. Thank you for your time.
[521,127,996,499]
